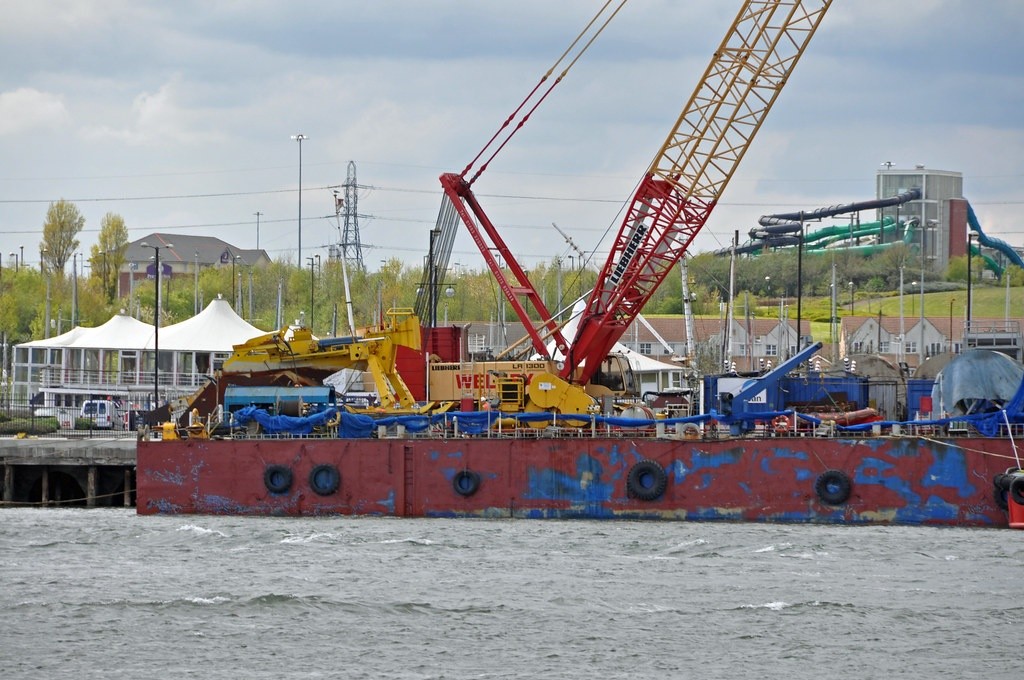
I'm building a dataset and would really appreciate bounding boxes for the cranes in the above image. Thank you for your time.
[408,0,832,429]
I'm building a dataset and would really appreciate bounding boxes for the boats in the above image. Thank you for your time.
[130,0,1024,529]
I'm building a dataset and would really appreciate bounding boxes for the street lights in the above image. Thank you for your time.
[9,252,19,272]
[522,269,530,316]
[288,132,309,272]
[911,280,917,316]
[849,281,856,314]
[568,254,575,271]
[765,275,772,317]
[312,254,321,286]
[39,248,49,277]
[100,250,107,300]
[380,259,387,271]
[138,241,175,438]
[18,245,28,267]
[493,253,502,267]
[306,256,314,329]
[231,254,243,309]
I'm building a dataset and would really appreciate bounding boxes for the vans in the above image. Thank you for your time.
[81,399,118,429]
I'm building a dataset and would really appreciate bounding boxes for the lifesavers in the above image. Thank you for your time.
[992,484,1024,511]
[322,407,341,428]
[308,463,341,497]
[264,463,294,494]
[453,469,481,496]
[815,469,851,505]
[773,414,791,433]
[627,458,668,502]
[681,422,701,440]
